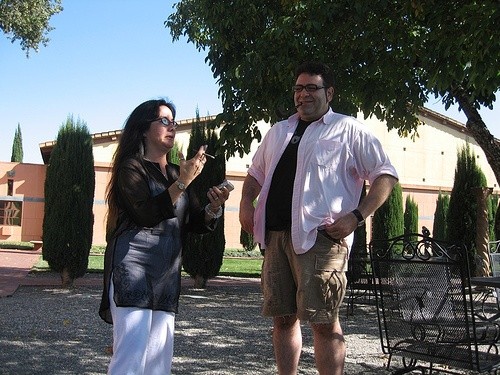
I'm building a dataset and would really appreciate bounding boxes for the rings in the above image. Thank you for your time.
[215,197,218,201]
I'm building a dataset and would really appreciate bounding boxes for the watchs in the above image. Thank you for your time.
[352,209,365,228]
[175,180,186,191]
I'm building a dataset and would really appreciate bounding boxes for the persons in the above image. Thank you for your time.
[239,64,399,375]
[98,100,229,375]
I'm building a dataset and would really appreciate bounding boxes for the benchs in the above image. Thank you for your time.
[360,273,436,279]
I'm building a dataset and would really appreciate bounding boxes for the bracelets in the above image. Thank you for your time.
[206,204,223,218]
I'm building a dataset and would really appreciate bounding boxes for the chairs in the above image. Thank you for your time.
[347,252,377,316]
[369,227,500,375]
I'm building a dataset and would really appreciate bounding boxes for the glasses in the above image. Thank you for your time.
[151,117,179,129]
[291,84,328,92]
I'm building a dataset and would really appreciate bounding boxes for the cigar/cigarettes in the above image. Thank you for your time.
[295,102,303,108]
[193,149,215,160]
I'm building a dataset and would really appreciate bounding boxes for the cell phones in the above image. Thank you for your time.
[216,179,234,192]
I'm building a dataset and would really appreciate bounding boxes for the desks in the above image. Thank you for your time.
[466,275,500,310]
